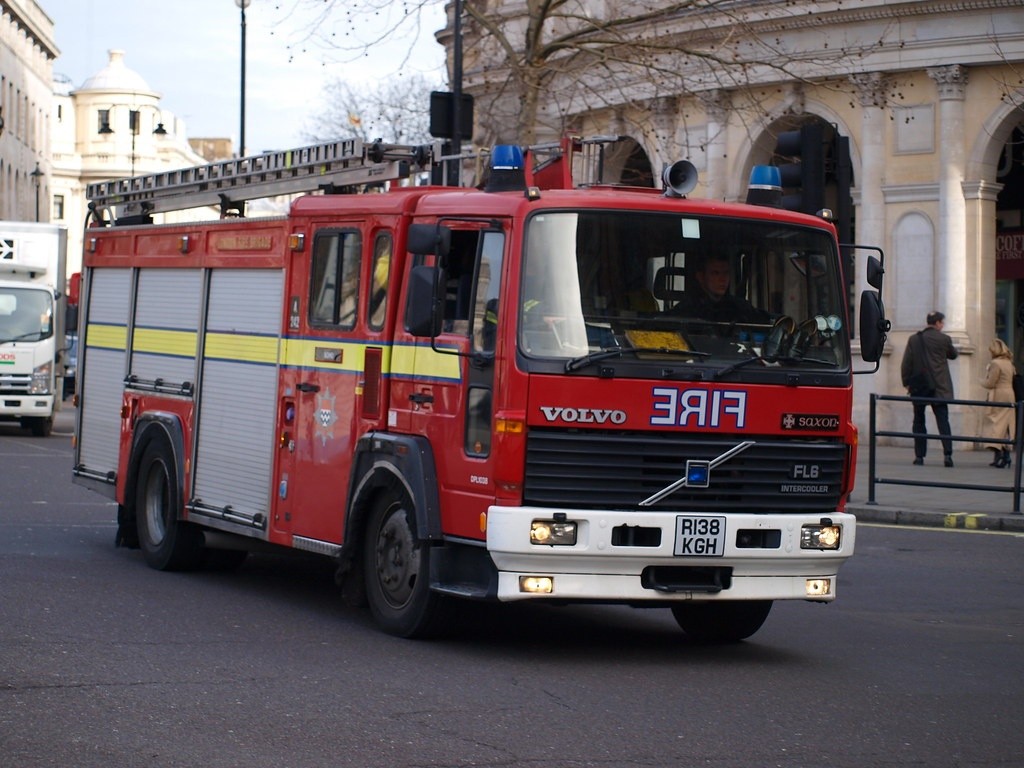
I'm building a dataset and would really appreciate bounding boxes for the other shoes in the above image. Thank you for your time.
[943,455,954,468]
[911,456,923,465]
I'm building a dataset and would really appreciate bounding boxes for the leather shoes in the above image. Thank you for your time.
[787,318,818,362]
[761,314,796,363]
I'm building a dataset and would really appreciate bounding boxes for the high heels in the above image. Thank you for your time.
[995,456,1011,468]
[989,455,1002,466]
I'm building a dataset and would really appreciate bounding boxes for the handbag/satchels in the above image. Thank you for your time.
[906,330,937,406]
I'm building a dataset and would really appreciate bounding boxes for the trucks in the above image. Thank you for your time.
[0,220,67,437]
[65,300,80,401]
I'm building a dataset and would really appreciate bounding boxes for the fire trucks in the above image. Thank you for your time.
[73,138,892,640]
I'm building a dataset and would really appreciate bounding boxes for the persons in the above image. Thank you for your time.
[979,339,1016,469]
[901,311,958,467]
[484,247,568,353]
[673,254,796,340]
[12,297,41,339]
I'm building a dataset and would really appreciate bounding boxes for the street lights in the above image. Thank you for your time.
[235,0,252,157]
